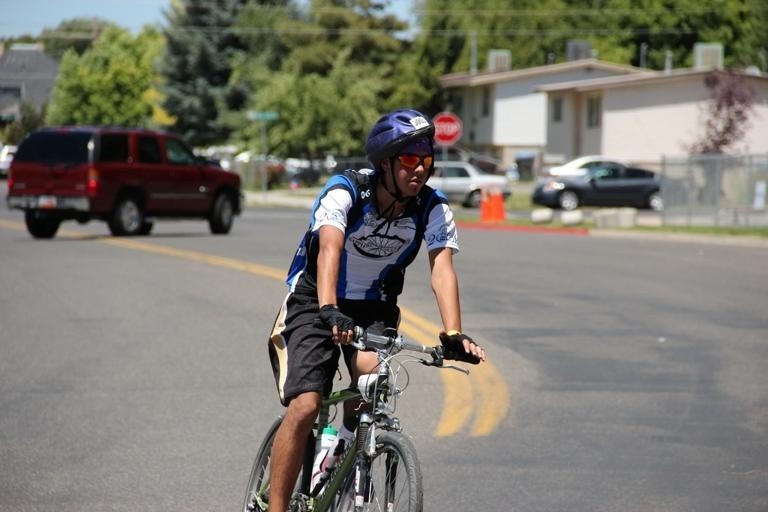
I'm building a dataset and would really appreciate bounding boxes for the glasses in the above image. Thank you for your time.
[391,152,436,169]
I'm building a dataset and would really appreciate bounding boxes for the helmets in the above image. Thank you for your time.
[364,107,435,171]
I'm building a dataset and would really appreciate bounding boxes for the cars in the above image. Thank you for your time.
[426,158,515,210]
[550,153,636,181]
[431,141,509,176]
[530,162,664,209]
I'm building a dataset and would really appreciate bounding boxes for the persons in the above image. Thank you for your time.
[266,110,488,512]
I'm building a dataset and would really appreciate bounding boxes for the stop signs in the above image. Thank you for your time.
[431,111,465,146]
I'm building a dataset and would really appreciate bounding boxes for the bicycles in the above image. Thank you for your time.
[237,320,484,512]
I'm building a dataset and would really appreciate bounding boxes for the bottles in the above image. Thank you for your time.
[308,423,340,498]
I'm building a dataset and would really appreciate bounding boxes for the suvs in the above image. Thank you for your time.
[5,122,245,239]
